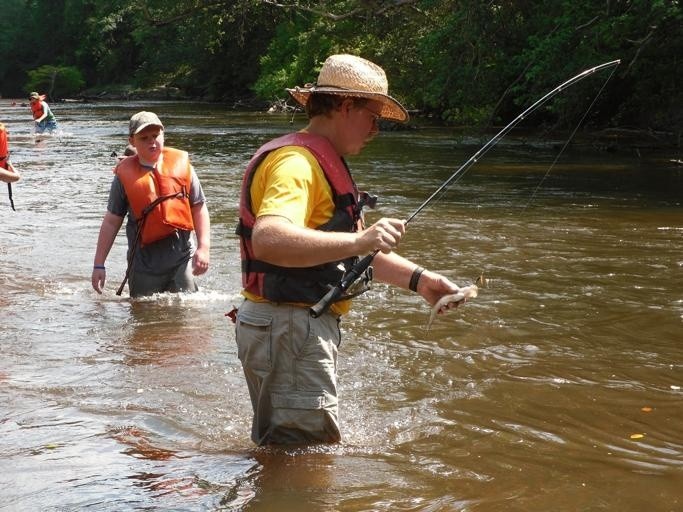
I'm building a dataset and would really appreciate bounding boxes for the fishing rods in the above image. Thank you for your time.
[309,59,621,321]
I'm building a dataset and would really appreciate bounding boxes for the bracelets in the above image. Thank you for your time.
[94,266,105,270]
[409,268,425,292]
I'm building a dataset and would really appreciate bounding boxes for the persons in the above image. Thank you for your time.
[234,56,466,448]
[11,97,16,106]
[91,112,211,299]
[0,122,20,183]
[21,102,27,107]
[29,92,58,145]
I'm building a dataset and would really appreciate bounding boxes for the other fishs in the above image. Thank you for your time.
[426,283,479,333]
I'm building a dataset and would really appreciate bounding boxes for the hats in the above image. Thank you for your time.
[29,92,39,99]
[129,111,164,135]
[284,53,410,124]
